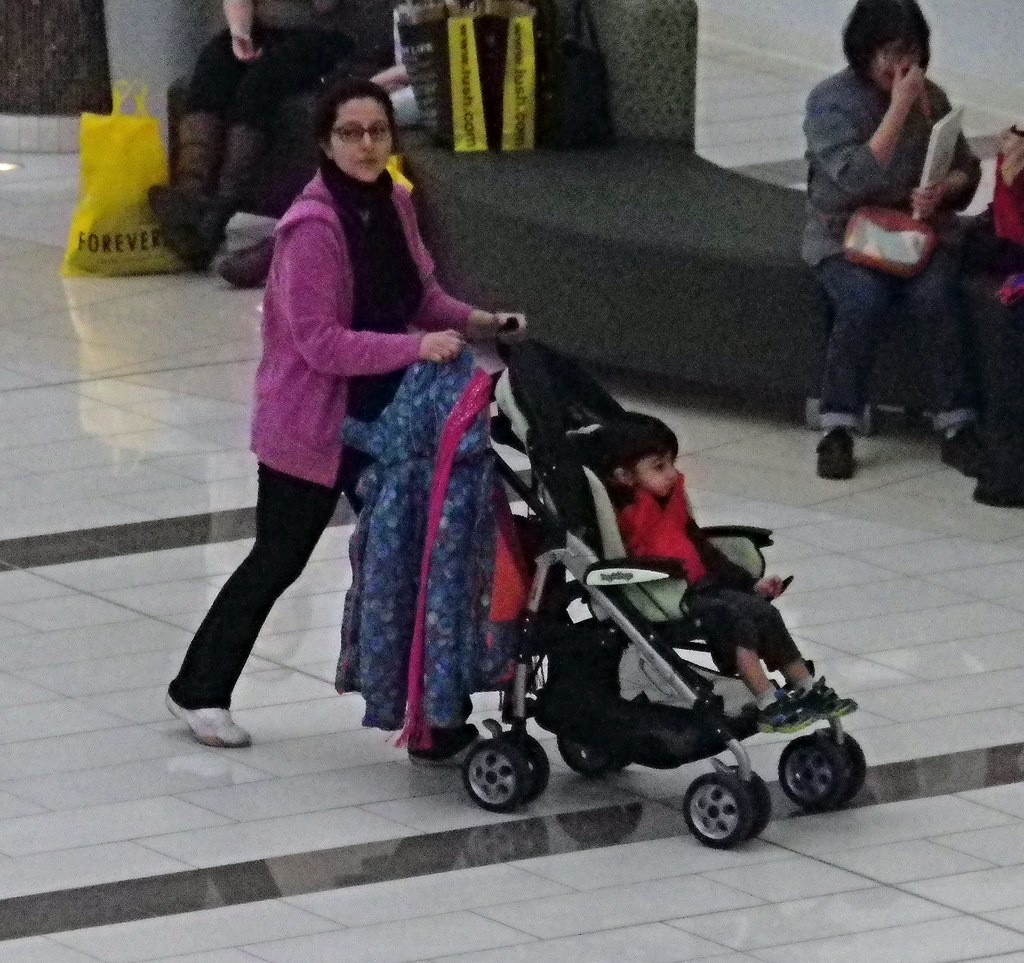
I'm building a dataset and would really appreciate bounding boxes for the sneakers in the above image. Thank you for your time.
[163,693,251,747]
[757,690,816,732]
[804,682,857,720]
[409,736,486,767]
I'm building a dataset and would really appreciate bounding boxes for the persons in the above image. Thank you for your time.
[589,412,857,734]
[802,0,1024,509]
[148,0,425,285]
[163,77,525,748]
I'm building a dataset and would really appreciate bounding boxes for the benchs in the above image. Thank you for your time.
[395,0,822,406]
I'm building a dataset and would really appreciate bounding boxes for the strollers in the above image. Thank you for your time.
[420,317,867,849]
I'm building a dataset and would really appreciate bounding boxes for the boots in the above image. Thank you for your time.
[148,113,222,230]
[162,126,267,274]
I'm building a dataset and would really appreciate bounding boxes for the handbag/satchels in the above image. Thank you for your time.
[61,78,183,278]
[844,205,936,277]
[397,0,610,156]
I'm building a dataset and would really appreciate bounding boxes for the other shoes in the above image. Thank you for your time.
[971,457,1024,506]
[815,428,856,478]
[219,237,279,290]
[941,426,994,477]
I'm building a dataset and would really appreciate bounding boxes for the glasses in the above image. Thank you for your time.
[333,124,390,143]
